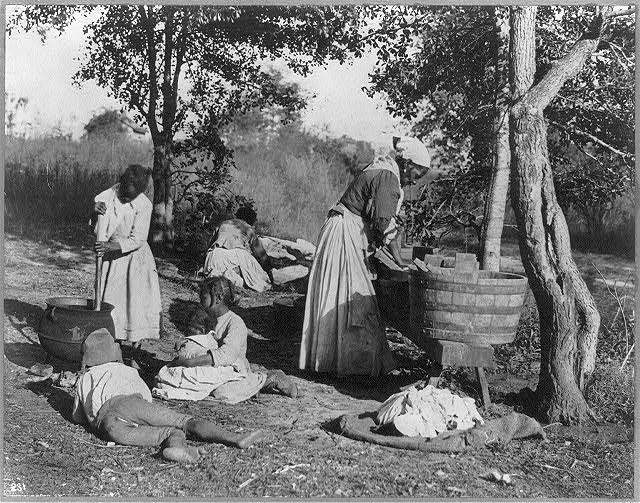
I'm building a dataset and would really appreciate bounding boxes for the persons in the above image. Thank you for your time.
[300,135,431,387]
[152,275,297,404]
[202,206,275,291]
[169,296,219,366]
[70,327,270,466]
[89,162,163,344]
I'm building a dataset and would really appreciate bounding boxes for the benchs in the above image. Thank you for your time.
[389,314,496,408]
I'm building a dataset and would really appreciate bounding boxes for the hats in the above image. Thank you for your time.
[78,329,124,369]
[393,134,432,171]
